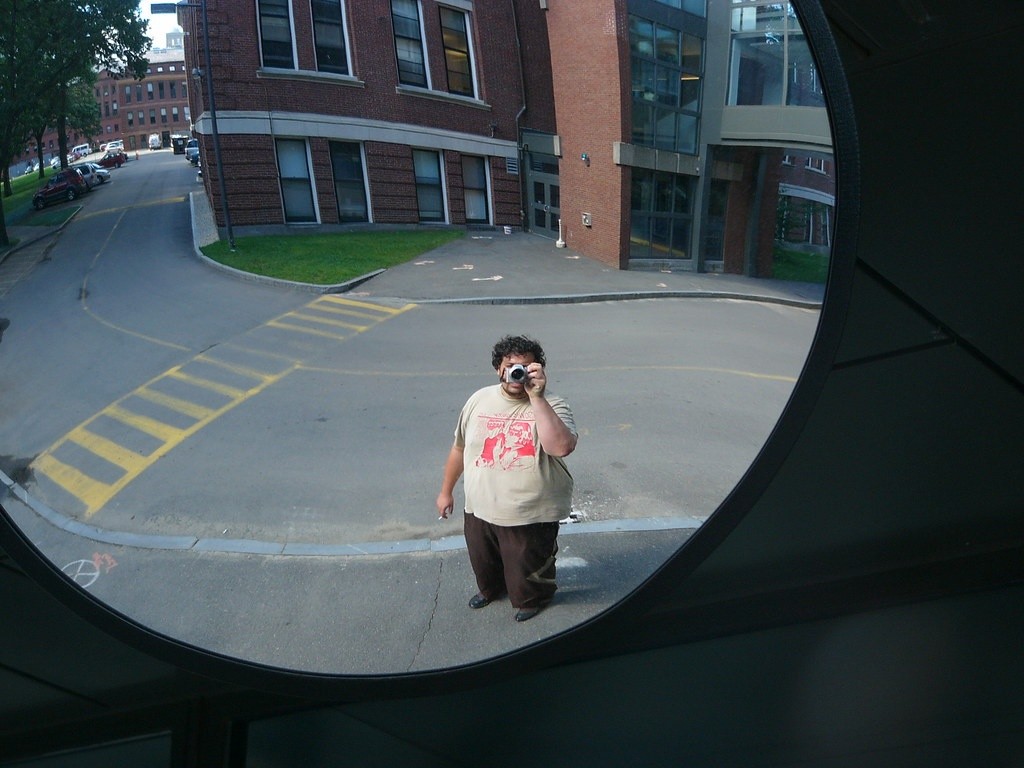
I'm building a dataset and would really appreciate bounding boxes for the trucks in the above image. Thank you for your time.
[148,134,162,150]
[171,135,189,155]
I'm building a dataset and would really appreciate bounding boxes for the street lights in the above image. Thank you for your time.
[151,0,236,255]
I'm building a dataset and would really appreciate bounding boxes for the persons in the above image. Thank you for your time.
[433,335,578,622]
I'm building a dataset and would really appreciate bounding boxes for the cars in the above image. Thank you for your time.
[24,162,42,175]
[98,148,126,169]
[100,140,125,154]
[50,154,72,169]
[91,162,110,185]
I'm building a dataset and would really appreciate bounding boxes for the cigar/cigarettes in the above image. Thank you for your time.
[438,511,447,519]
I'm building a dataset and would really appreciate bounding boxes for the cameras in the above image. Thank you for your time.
[505,364,531,383]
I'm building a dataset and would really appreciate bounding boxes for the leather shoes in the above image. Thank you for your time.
[468,590,508,609]
[515,607,547,621]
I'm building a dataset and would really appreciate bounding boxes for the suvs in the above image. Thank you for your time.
[68,163,99,193]
[32,167,87,211]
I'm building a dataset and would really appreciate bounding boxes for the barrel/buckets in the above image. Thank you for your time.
[504,226,512,235]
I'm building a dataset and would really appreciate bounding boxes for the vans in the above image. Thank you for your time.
[185,138,199,160]
[71,143,89,157]
